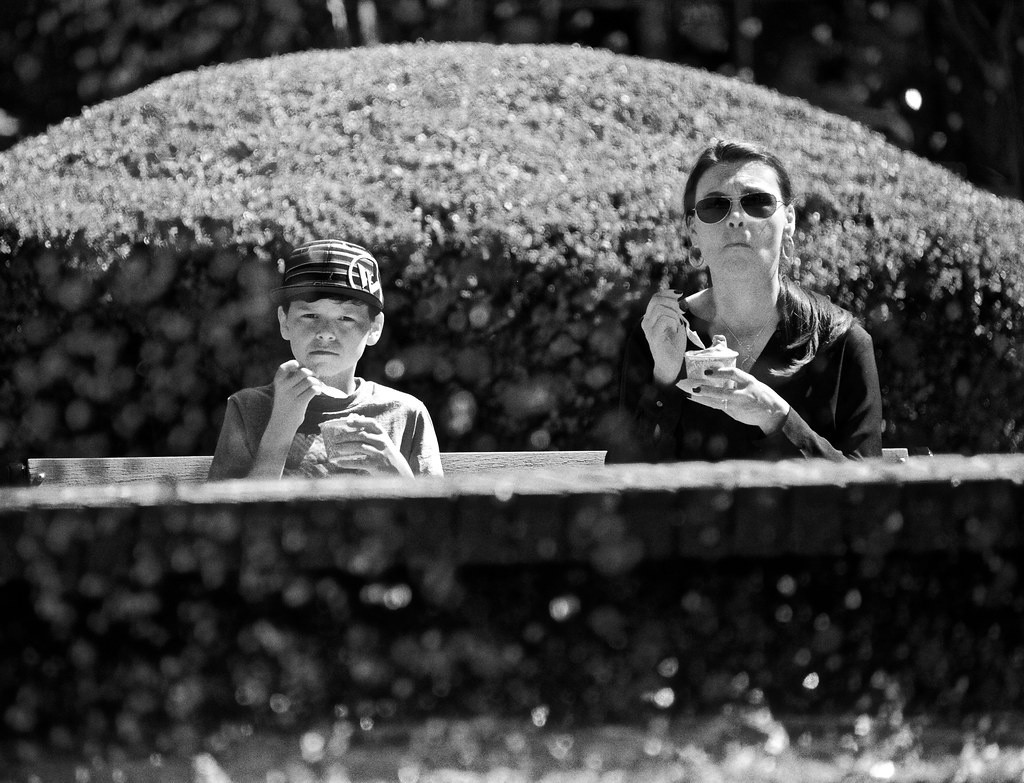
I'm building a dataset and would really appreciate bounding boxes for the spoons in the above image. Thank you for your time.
[682,314,706,349]
[322,386,349,399]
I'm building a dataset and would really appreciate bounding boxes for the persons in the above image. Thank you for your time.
[603,141,886,464]
[207,238,447,482]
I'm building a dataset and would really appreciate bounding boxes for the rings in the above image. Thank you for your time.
[724,400,728,410]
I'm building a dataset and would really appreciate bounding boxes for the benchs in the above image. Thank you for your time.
[10,447,908,483]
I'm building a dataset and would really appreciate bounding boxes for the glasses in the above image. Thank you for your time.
[690,193,787,224]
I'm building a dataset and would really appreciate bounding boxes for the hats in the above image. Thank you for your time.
[270,238,385,309]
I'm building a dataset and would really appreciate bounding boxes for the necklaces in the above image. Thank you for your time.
[717,310,775,372]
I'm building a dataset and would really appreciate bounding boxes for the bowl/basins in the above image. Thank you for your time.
[684,351,739,380]
[318,418,367,464]
